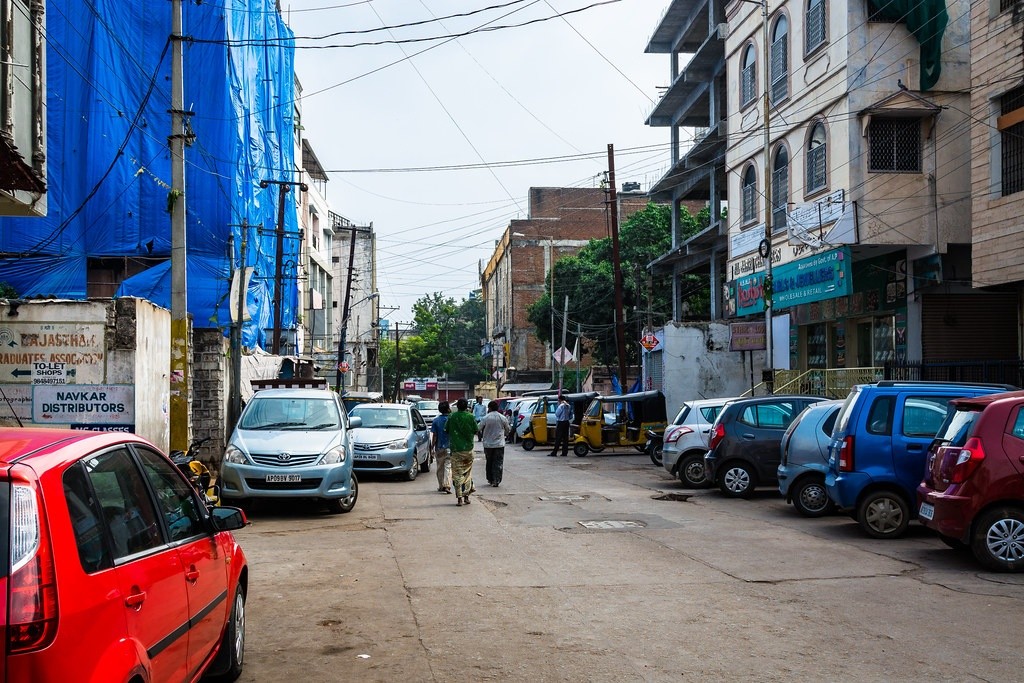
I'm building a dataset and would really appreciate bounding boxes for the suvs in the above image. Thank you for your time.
[824,380,1023,539]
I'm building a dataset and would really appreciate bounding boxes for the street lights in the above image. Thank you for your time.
[470,295,498,389]
[354,315,382,392]
[513,232,555,383]
[336,291,381,393]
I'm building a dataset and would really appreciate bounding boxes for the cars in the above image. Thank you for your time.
[0,427,248,683]
[348,403,436,481]
[916,390,1024,573]
[221,388,362,513]
[777,399,847,518]
[703,395,835,499]
[403,395,443,426]
[662,396,792,490]
[450,397,616,438]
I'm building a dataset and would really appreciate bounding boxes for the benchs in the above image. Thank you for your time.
[569,420,582,428]
[627,419,641,431]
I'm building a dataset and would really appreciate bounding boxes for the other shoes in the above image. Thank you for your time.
[547,453,557,456]
[455,502,463,506]
[464,499,471,504]
[445,488,452,494]
[489,481,499,487]
[438,488,444,492]
[560,453,567,456]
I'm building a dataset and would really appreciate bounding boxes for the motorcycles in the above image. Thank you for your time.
[521,391,605,451]
[573,389,668,457]
[169,437,220,505]
[643,430,663,467]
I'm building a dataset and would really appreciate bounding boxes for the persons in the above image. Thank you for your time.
[477,401,511,487]
[505,409,518,443]
[473,396,486,442]
[430,401,452,494]
[548,396,573,457]
[443,398,478,506]
